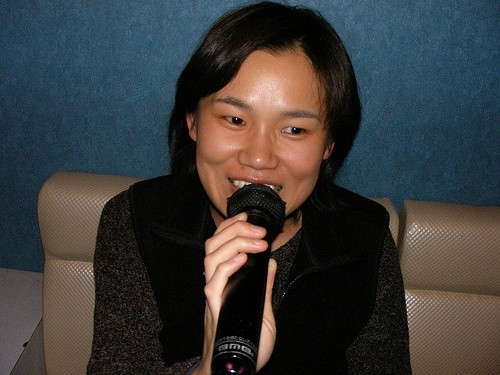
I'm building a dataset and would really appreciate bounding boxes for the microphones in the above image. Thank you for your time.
[211,184,287,375]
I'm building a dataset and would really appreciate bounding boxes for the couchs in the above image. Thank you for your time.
[6,172,500,375]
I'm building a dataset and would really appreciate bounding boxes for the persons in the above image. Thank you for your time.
[87,0,413,375]
